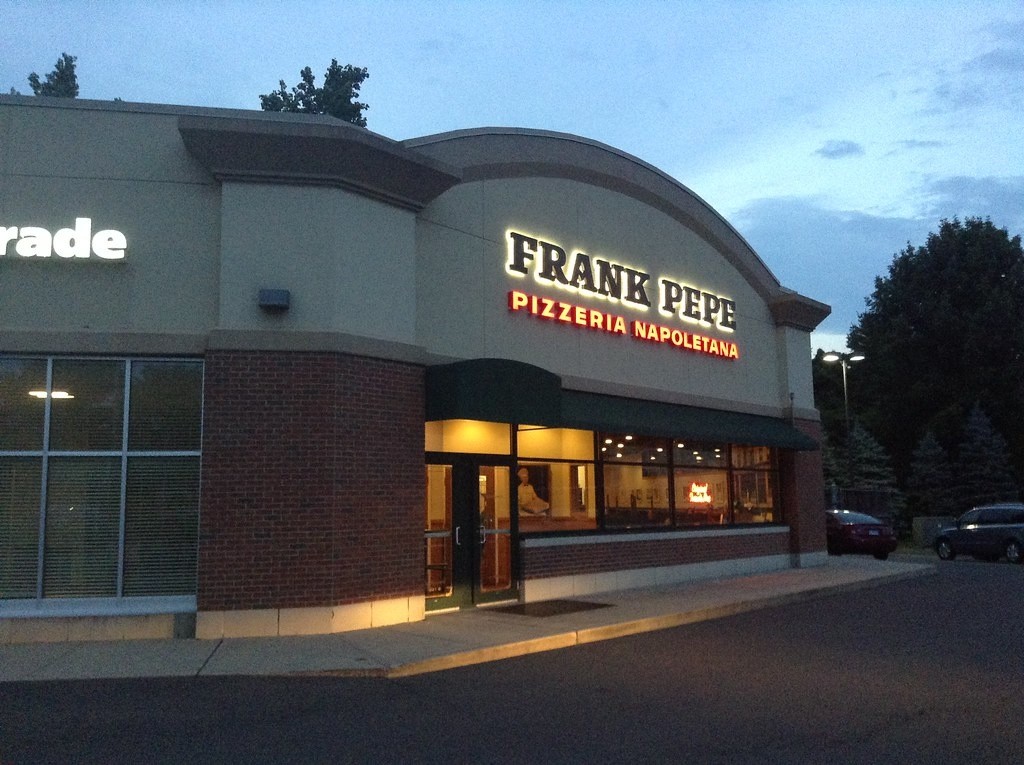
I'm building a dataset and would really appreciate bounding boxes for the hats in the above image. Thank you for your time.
[518,469,528,477]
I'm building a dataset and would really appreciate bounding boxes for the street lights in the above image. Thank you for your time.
[822,354,866,489]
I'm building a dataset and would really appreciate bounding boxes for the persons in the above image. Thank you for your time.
[517,467,549,517]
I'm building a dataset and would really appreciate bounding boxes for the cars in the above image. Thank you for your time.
[929,503,1024,562]
[824,507,899,561]
